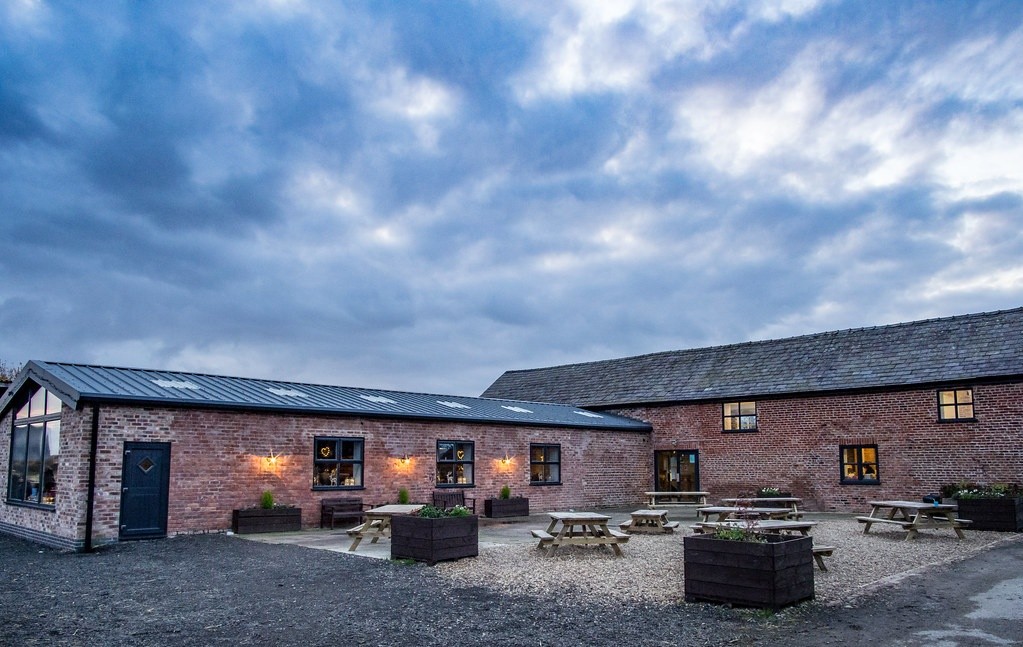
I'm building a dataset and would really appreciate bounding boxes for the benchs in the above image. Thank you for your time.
[619,520,680,529]
[347,521,392,537]
[433,490,475,514]
[643,502,713,509]
[689,511,836,554]
[531,529,630,545]
[320,497,374,530]
[854,515,971,530]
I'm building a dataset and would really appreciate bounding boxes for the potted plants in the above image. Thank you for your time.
[940,479,1023,532]
[756,487,792,520]
[389,487,529,567]
[683,493,815,611]
[231,491,301,534]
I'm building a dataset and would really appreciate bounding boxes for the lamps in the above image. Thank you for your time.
[502,454,509,463]
[402,454,409,463]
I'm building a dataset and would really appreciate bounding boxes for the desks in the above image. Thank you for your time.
[722,497,803,521]
[536,512,623,557]
[695,507,793,522]
[350,505,426,551]
[626,510,673,534]
[863,501,966,541]
[645,492,710,522]
[695,520,827,571]
